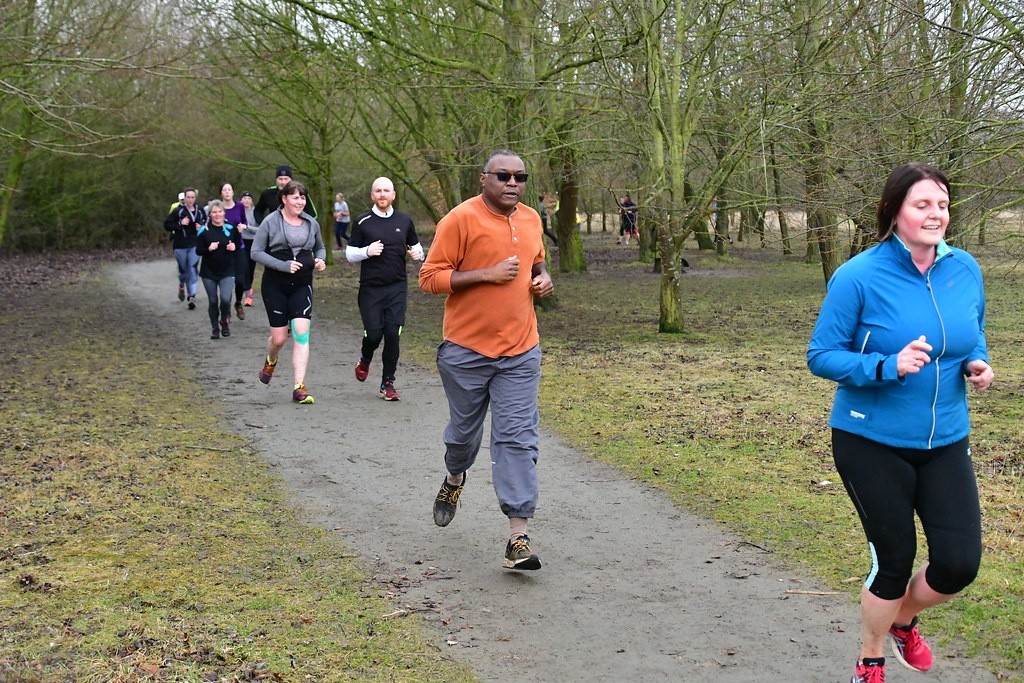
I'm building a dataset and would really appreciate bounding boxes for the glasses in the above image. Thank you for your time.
[484,171,529,183]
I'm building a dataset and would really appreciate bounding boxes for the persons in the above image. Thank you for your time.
[220,182,248,322]
[333,191,350,249]
[253,165,319,227]
[803,159,996,683]
[162,188,212,310]
[418,148,557,570]
[345,176,425,402]
[250,180,326,405]
[554,190,581,232]
[615,194,640,246]
[194,200,242,339]
[539,196,557,247]
[238,193,261,307]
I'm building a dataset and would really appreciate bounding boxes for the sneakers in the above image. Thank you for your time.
[380,382,400,401]
[888,615,933,672]
[178,285,185,301]
[235,302,245,320]
[188,300,196,311]
[244,297,253,305]
[249,288,254,297]
[433,470,468,527]
[293,384,314,404]
[259,354,278,385]
[219,319,230,336]
[210,327,220,339]
[502,533,542,570]
[355,352,372,383]
[851,654,886,683]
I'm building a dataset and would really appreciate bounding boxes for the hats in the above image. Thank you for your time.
[179,192,184,200]
[240,191,252,199]
[276,165,292,178]
